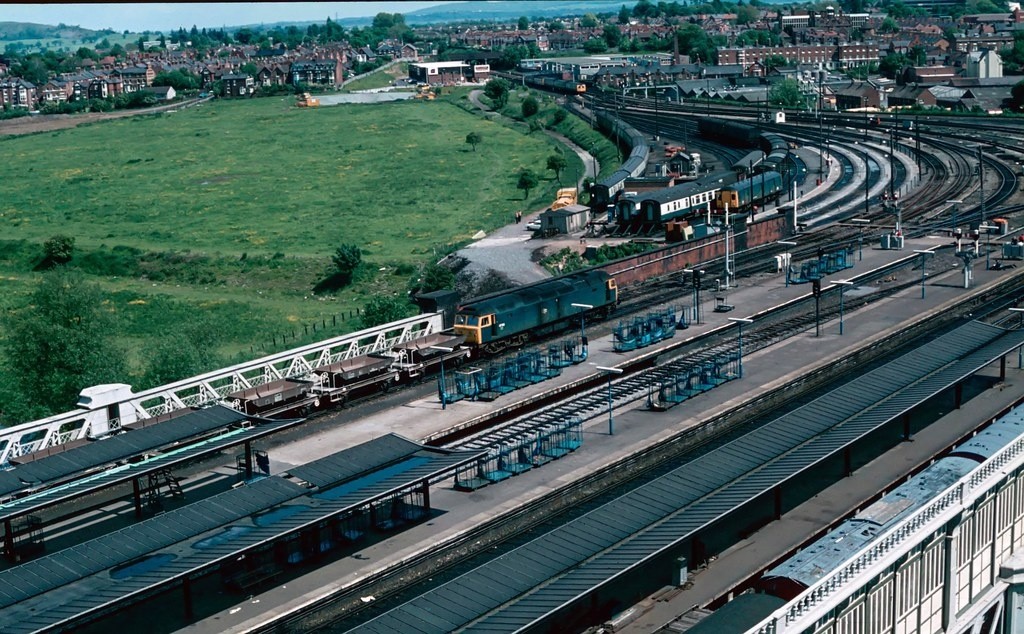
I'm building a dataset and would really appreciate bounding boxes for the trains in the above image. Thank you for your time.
[0,269,618,523]
[585,109,650,209]
[614,147,766,224]
[640,114,791,226]
[715,170,785,210]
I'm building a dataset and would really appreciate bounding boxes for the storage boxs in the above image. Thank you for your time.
[298,92,320,107]
[414,85,436,99]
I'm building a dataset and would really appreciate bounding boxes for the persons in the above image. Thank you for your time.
[893,192,898,207]
[1019,234,1024,242]
[882,191,888,205]
[516,210,521,224]
[994,260,1004,270]
[817,247,824,260]
[1011,236,1018,245]
[890,229,902,243]
